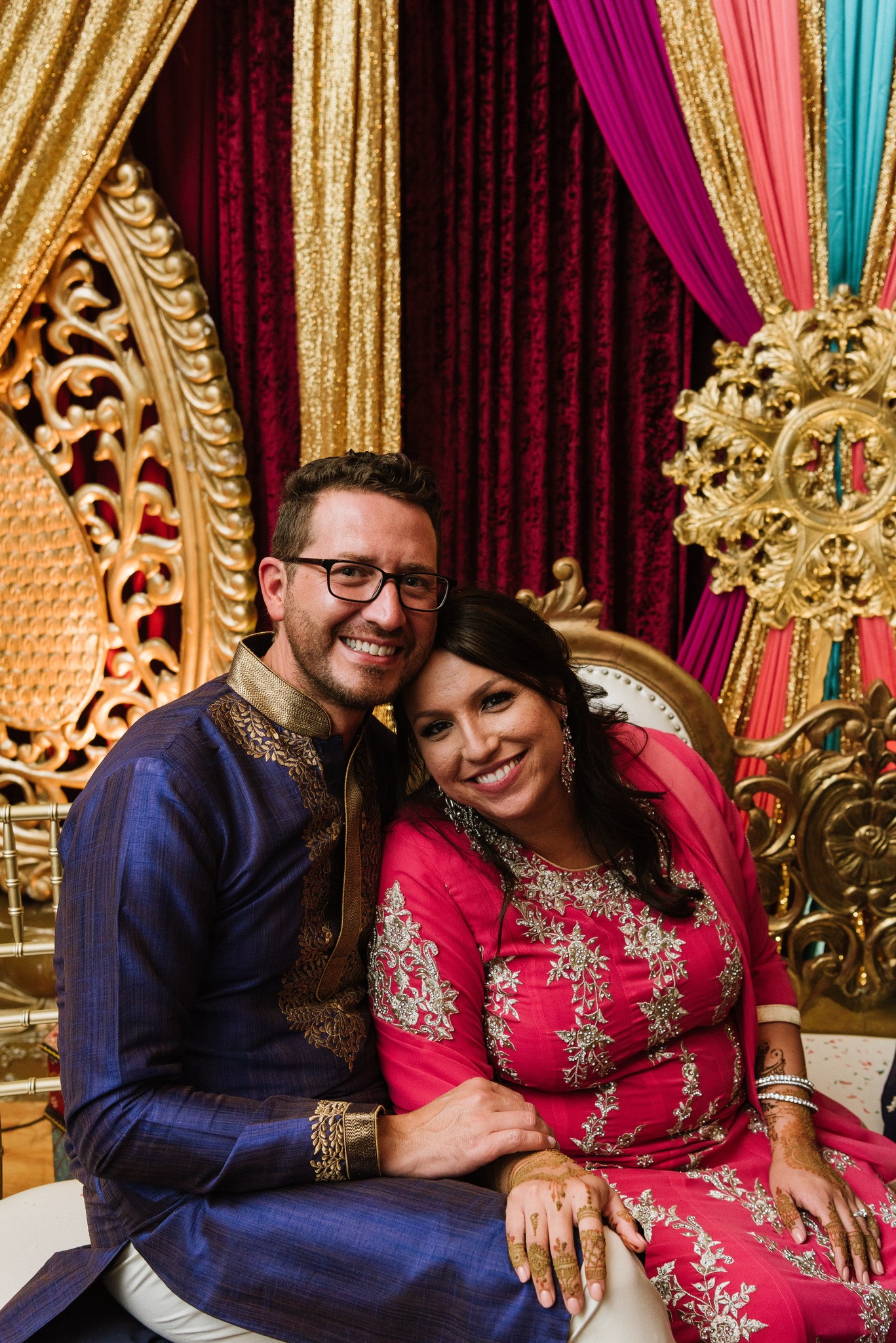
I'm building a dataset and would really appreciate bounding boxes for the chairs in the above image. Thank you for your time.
[717,675,896,1140]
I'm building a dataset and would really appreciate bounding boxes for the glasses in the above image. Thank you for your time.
[280,555,451,612]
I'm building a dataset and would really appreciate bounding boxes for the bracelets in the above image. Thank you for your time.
[755,1074,815,1100]
[755,1093,820,1115]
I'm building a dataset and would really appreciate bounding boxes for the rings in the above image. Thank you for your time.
[852,1208,868,1218]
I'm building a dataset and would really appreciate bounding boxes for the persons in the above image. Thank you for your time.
[52,453,681,1343]
[366,587,896,1342]
